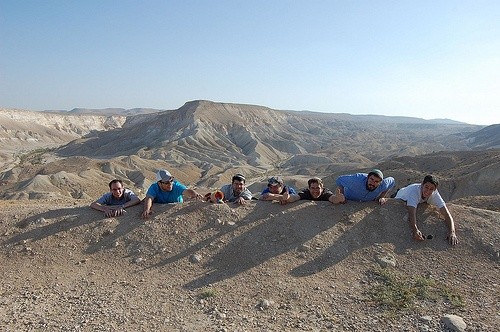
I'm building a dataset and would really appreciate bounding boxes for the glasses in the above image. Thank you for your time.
[161,179,173,184]
[421,234,433,239]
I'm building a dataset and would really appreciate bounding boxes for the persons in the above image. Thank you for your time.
[220,173,253,205]
[390,175,458,245]
[90,179,140,218]
[283,178,344,204]
[335,169,396,206]
[259,176,300,205]
[141,169,204,218]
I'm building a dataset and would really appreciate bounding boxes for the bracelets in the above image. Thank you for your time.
[120,204,125,209]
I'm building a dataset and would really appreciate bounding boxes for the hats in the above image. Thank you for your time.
[156,169,174,181]
[368,169,383,181]
[268,177,283,186]
